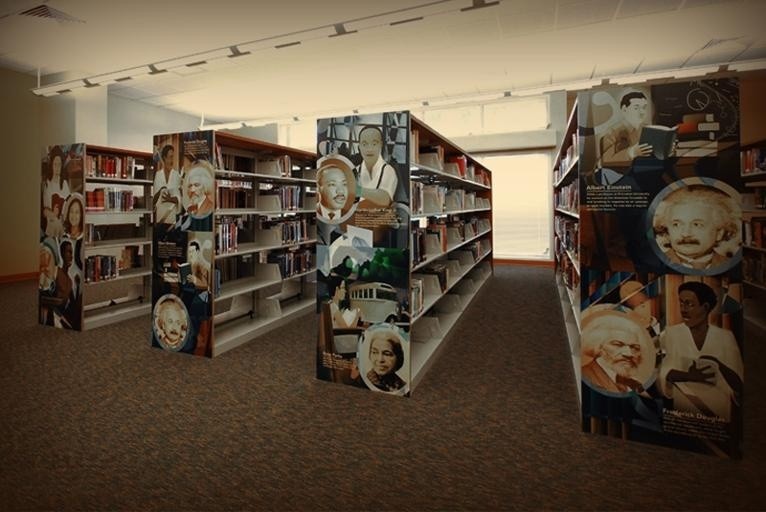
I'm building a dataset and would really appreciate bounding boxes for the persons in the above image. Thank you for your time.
[600,90,680,164]
[152,142,214,349]
[580,316,648,395]
[655,186,741,270]
[657,280,742,420]
[317,121,410,380]
[40,145,82,332]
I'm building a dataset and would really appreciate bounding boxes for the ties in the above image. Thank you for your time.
[328,213,335,219]
[692,264,705,270]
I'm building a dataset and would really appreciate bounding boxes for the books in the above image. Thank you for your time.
[739,145,765,289]
[407,128,492,317]
[553,132,582,299]
[81,145,153,323]
[630,113,720,161]
[214,142,319,301]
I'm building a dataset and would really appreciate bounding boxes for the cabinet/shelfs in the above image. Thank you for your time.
[551,75,746,464]
[312,107,494,400]
[40,141,151,332]
[148,127,318,361]
